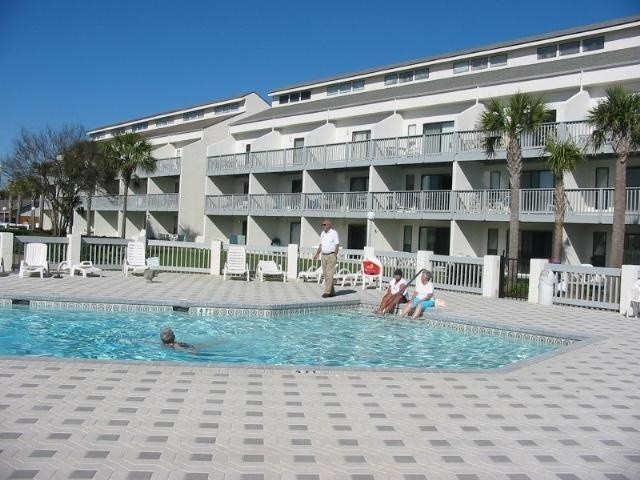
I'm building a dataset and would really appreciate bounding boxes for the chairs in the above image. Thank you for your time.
[19,243,49,278]
[361,258,383,290]
[123,242,152,277]
[255,260,286,283]
[297,263,338,284]
[224,244,250,282]
[322,262,374,288]
[59,261,103,278]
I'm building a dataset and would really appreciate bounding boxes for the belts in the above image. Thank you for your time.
[322,252,334,255]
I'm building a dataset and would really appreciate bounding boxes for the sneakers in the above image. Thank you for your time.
[321,293,335,298]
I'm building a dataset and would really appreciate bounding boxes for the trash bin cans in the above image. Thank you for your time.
[271,238,280,255]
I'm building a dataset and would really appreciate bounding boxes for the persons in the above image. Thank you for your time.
[372,268,410,315]
[145,327,222,354]
[402,269,436,319]
[312,218,340,298]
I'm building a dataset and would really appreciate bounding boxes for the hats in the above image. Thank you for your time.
[160,327,173,341]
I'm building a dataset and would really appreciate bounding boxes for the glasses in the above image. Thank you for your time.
[322,224,328,226]
